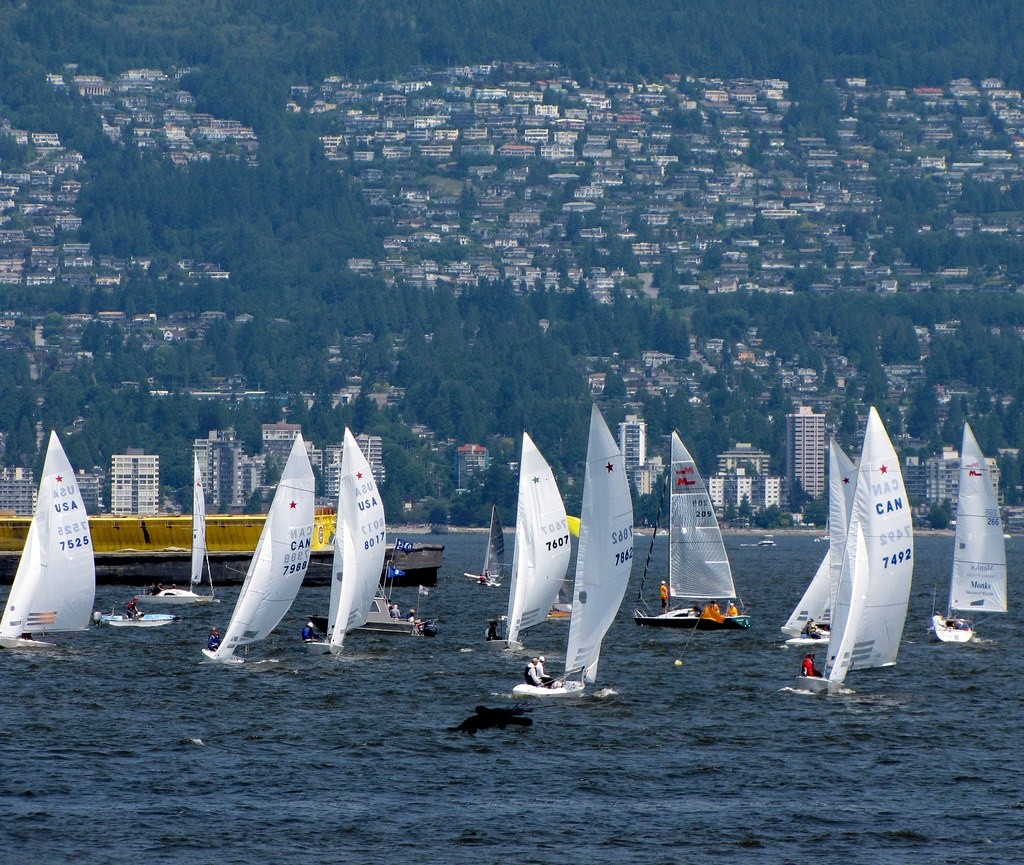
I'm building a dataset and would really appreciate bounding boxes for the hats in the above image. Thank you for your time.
[539,656,545,662]
[307,622,314,627]
[409,608,414,612]
[532,658,540,662]
[662,580,666,584]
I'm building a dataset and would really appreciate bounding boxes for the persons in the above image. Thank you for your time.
[485,569,491,580]
[660,580,668,613]
[525,656,553,687]
[933,611,947,630]
[127,598,138,618]
[802,655,823,677]
[302,622,319,643]
[390,604,401,618]
[487,626,502,641]
[710,601,719,612]
[958,622,970,630]
[723,603,738,616]
[690,606,702,617]
[208,628,220,651]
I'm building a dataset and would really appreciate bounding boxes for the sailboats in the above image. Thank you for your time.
[201,433,316,671]
[513,401,634,698]
[134,451,221,604]
[781,407,858,649]
[926,421,1008,642]
[0,431,95,651]
[633,428,751,629]
[464,504,505,587]
[795,406,914,693]
[305,425,387,654]
[480,427,580,652]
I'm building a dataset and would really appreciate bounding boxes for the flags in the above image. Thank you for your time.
[396,539,414,552]
[702,606,725,623]
[388,567,405,577]
[419,585,430,595]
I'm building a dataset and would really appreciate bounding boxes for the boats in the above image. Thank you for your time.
[0,509,445,587]
[814,535,830,542]
[93,607,180,628]
[307,538,438,637]
[758,539,774,546]
[431,524,448,534]
